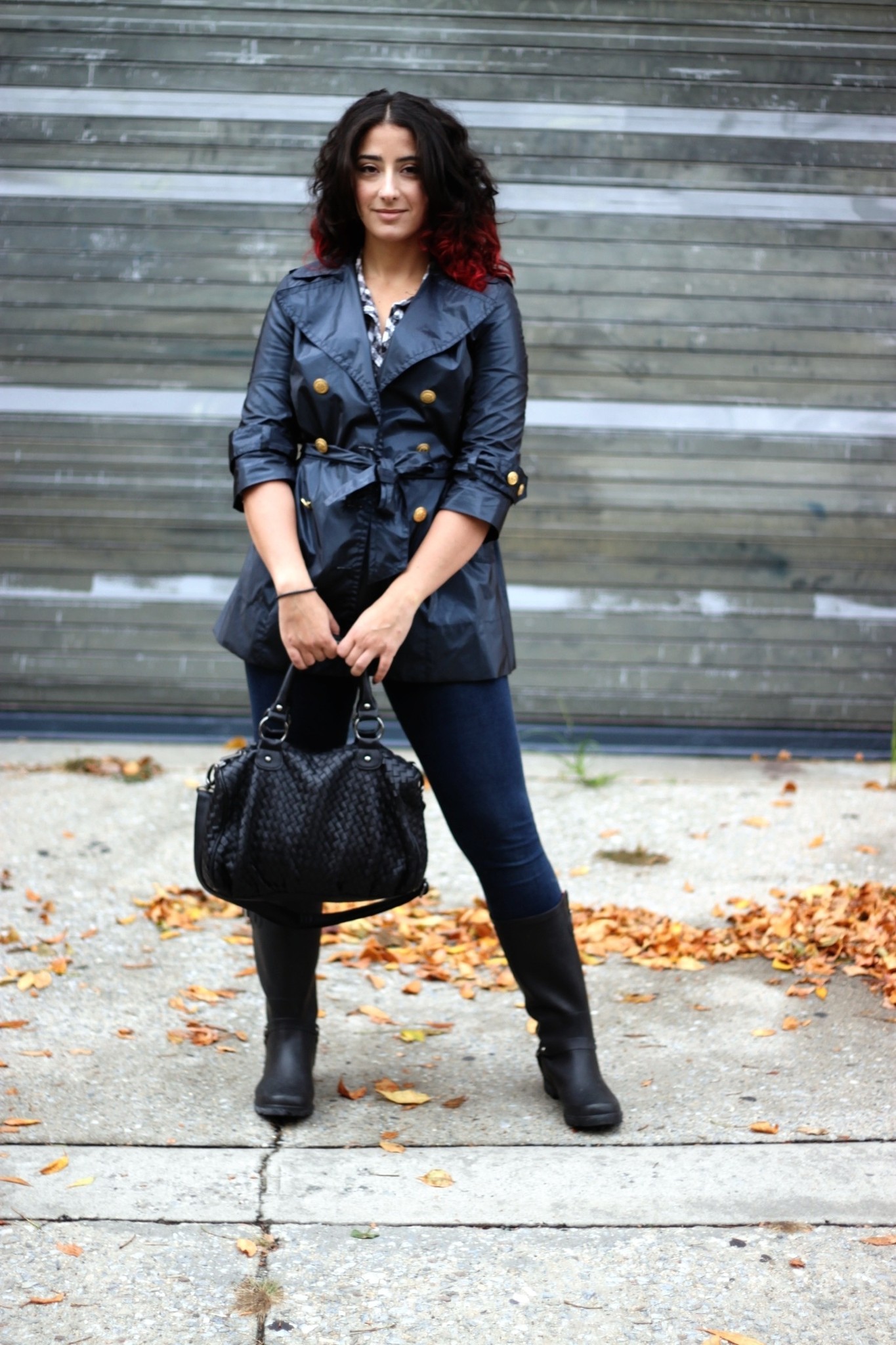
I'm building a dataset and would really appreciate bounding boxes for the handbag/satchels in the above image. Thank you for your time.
[191,641,430,924]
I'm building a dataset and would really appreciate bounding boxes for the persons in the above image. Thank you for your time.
[211,89,622,1135]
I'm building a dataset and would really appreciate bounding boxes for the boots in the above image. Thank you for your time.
[486,888,624,1131]
[251,915,323,1121]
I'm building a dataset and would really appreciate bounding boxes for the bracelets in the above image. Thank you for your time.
[274,588,320,598]
